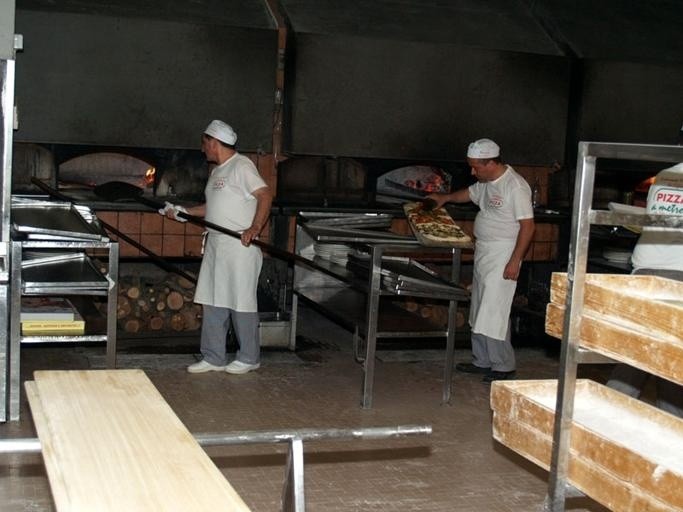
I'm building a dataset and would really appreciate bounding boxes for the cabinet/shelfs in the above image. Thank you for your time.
[9,228,119,420]
[290,218,461,409]
[545,140,683,512]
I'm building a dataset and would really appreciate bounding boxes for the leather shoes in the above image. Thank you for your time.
[455,363,491,374]
[481,371,518,385]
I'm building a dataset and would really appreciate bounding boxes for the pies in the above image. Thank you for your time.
[403,202,472,242]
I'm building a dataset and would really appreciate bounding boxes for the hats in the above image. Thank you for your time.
[204,120,238,146]
[467,138,500,159]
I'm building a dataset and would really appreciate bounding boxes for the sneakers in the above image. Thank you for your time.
[225,360,261,375]
[187,360,226,374]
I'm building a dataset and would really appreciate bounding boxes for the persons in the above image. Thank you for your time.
[425,138,535,381]
[166,119,273,375]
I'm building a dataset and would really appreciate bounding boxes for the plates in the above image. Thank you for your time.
[602,249,633,265]
[313,242,354,268]
